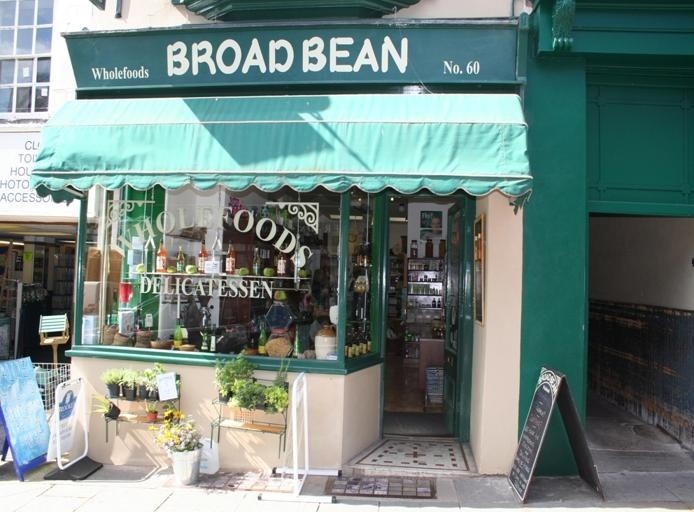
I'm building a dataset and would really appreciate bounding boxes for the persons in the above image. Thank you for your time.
[422,216,440,240]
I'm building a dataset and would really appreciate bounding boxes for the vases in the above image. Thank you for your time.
[166,443,204,486]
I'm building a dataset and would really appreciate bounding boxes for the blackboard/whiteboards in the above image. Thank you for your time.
[1,356,52,469]
[507,367,562,504]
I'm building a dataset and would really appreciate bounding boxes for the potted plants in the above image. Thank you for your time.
[90,364,182,422]
[214,357,290,413]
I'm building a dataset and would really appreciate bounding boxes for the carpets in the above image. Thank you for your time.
[323,476,438,499]
[355,438,470,472]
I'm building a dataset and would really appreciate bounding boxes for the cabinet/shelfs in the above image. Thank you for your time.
[0,240,77,359]
[105,376,182,446]
[208,377,292,461]
[320,253,447,392]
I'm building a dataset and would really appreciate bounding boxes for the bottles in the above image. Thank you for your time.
[355,241,370,268]
[173,317,183,351]
[225,240,236,274]
[257,323,268,356]
[292,323,303,358]
[314,324,337,361]
[210,230,223,274]
[407,237,447,308]
[155,238,167,273]
[252,247,261,276]
[197,240,206,274]
[176,246,185,273]
[201,323,216,352]
[355,296,364,321]
[344,324,371,359]
[109,289,118,325]
[277,246,287,277]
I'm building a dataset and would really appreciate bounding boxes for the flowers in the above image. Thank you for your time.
[148,409,203,453]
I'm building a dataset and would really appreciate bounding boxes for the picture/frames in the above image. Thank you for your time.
[472,213,486,328]
